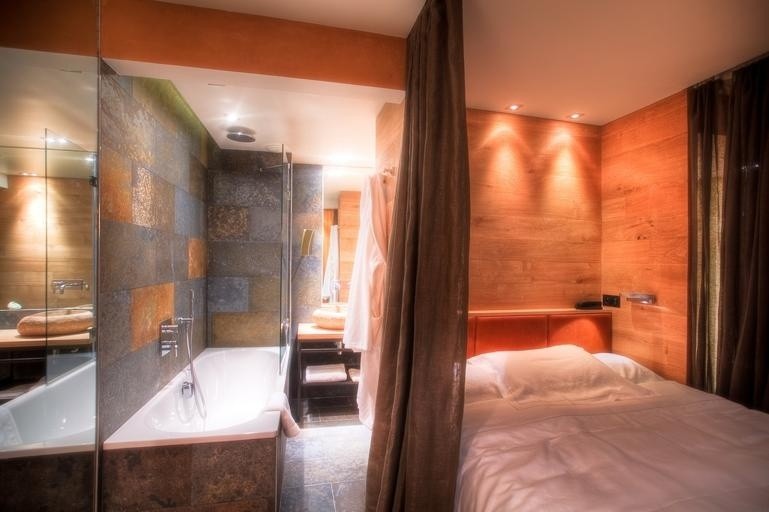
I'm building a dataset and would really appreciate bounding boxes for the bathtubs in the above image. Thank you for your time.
[103,344,288,450]
[0,359,96,459]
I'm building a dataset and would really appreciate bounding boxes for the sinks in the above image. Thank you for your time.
[17,309,93,337]
[312,305,348,330]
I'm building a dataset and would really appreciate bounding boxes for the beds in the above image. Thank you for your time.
[433,307,769,512]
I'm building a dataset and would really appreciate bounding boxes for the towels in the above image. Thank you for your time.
[305,362,347,383]
[349,367,362,383]
[264,390,301,437]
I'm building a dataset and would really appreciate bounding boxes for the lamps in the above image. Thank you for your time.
[290,228,313,279]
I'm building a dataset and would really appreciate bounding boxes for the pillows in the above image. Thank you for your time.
[438,344,657,405]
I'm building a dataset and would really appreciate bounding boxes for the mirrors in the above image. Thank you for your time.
[319,165,364,306]
[1,59,99,512]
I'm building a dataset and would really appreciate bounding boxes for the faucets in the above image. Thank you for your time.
[51,281,80,295]
[328,279,341,305]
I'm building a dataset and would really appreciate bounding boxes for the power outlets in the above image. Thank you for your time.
[602,294,620,307]
